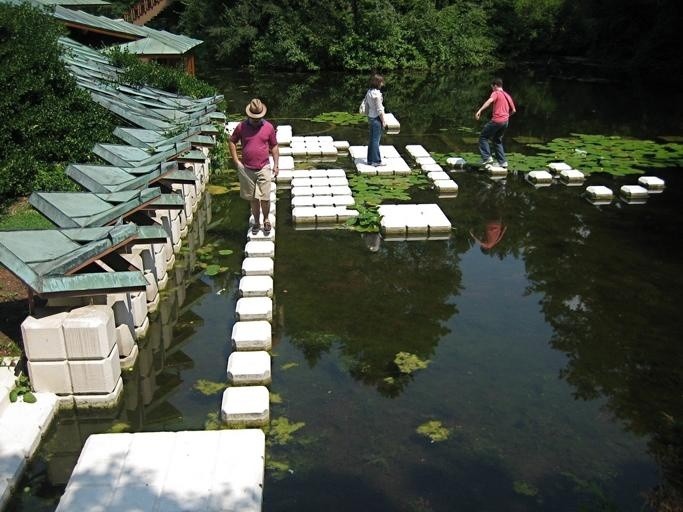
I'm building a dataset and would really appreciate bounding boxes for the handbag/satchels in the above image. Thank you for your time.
[359,96,369,116]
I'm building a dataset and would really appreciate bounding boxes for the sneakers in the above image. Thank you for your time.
[480,156,493,165]
[264,222,271,231]
[252,224,260,232]
[493,161,508,167]
[372,161,386,166]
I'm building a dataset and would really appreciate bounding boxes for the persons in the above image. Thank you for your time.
[474,78,516,167]
[360,231,381,251]
[229,98,280,233]
[366,74,386,166]
[469,216,507,254]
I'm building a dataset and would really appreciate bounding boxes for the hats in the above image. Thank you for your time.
[246,98,267,119]
